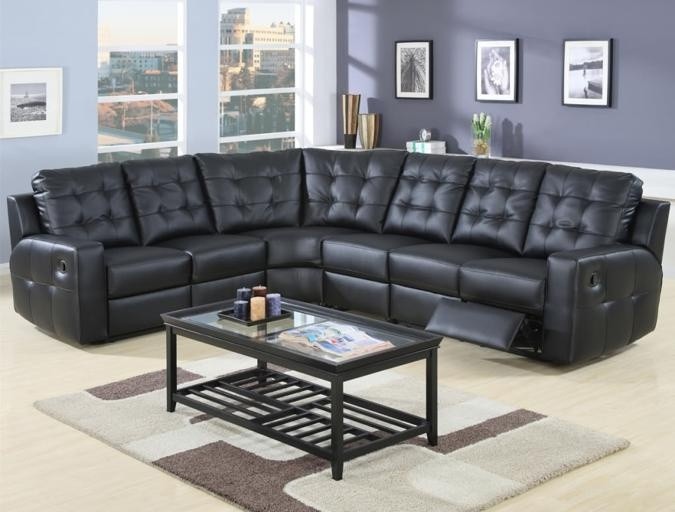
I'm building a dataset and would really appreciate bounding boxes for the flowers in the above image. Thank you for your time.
[471,113,492,145]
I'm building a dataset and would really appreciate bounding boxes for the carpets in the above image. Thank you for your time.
[34,351,632,512]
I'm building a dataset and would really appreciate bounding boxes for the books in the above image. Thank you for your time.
[276,320,396,358]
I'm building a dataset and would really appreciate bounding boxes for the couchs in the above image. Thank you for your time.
[6,149,671,375]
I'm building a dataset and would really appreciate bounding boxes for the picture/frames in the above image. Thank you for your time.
[2,67,64,138]
[394,40,433,100]
[560,38,613,110]
[474,38,519,105]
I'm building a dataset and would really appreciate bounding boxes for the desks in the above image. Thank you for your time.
[310,145,674,273]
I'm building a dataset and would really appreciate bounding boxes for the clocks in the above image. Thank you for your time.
[418,128,432,141]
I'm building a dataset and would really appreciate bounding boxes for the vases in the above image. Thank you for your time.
[472,139,490,158]
[341,94,360,149]
[357,112,380,148]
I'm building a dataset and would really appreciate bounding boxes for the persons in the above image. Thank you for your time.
[483,48,510,95]
